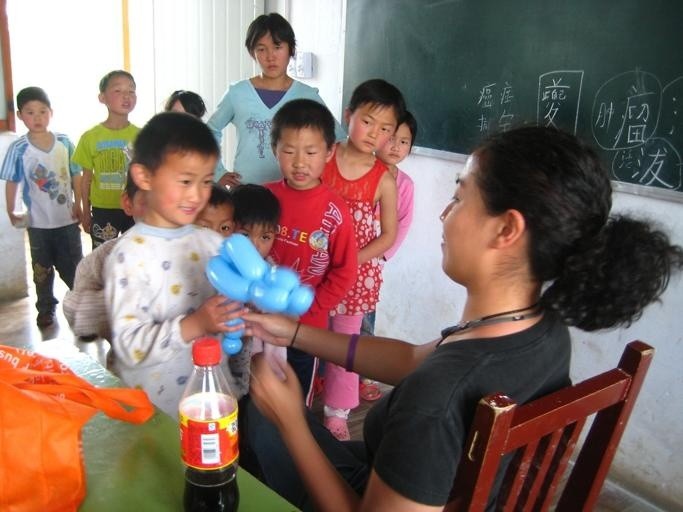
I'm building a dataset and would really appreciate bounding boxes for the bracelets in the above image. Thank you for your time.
[287,321,303,348]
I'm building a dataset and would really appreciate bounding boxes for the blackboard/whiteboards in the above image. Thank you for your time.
[341,0,682,204]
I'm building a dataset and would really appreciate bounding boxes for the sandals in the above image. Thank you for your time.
[356,383,383,402]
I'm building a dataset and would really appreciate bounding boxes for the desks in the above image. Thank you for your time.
[10,337,313,509]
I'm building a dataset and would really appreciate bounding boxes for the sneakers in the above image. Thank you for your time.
[34,306,57,327]
[324,416,351,441]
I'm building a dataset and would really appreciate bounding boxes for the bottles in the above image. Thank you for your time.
[178,338,241,511]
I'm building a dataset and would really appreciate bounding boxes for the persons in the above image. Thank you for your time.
[0,86,85,345]
[304,78,407,443]
[164,91,206,122]
[101,111,237,425]
[260,98,359,397]
[241,125,681,511]
[192,182,237,239]
[204,12,346,187]
[62,159,145,374]
[228,182,282,471]
[71,70,143,253]
[313,108,416,401]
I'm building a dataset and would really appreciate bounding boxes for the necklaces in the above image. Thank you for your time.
[440,307,548,336]
[435,299,542,348]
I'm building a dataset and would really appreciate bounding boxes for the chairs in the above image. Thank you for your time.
[440,338,656,509]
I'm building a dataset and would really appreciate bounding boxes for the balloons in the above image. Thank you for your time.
[205,232,313,356]
